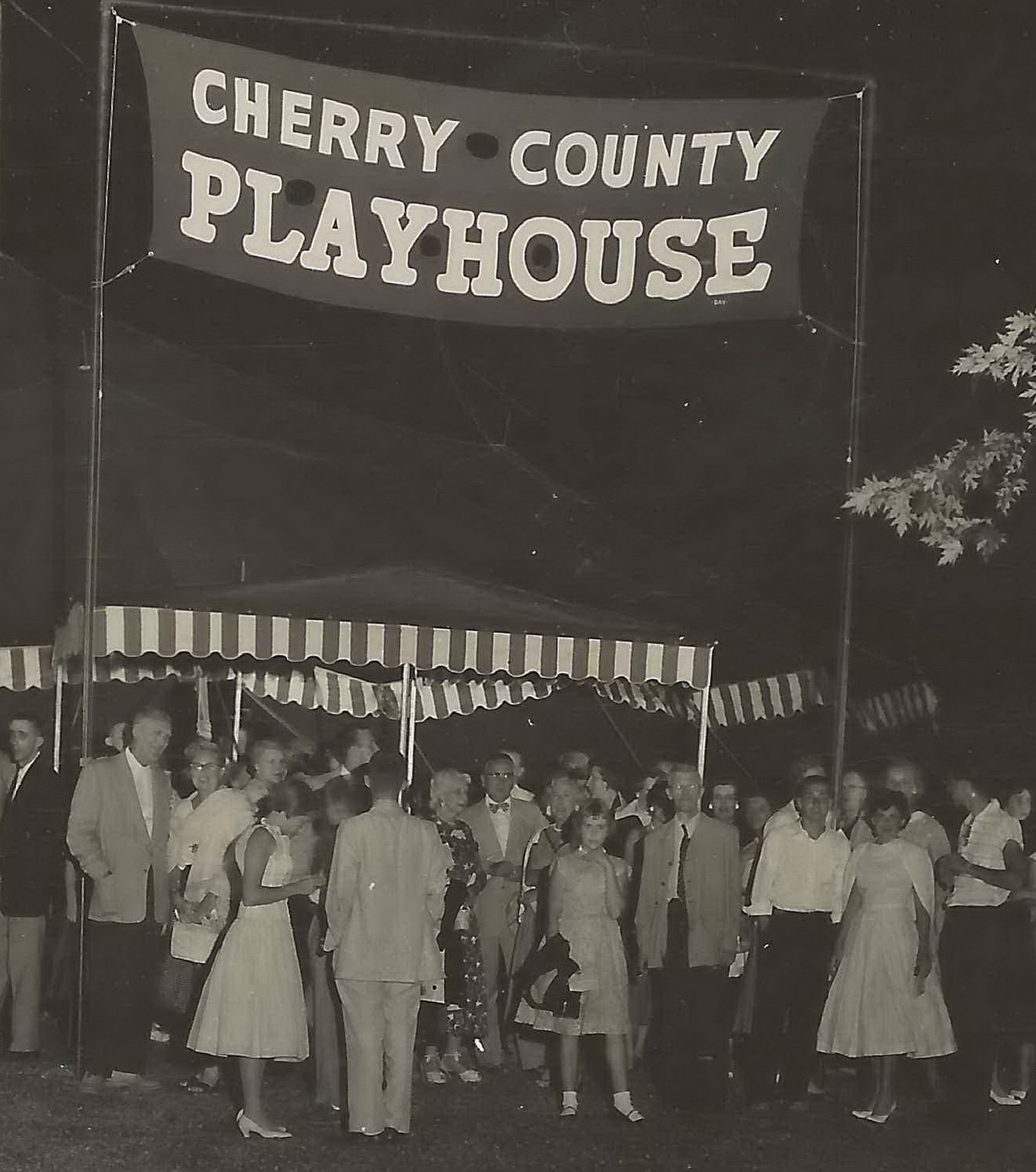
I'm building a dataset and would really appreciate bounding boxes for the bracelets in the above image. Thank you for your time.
[170,888,182,894]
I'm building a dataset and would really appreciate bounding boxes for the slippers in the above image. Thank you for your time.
[179,1074,222,1093]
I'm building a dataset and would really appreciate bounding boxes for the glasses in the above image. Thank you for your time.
[486,771,515,780]
[841,784,867,790]
[193,762,224,771]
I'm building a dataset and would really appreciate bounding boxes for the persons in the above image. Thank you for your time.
[515,798,649,1128]
[814,787,957,1125]
[185,779,328,1141]
[0,706,1036,1141]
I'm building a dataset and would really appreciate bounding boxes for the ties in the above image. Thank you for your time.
[677,824,691,901]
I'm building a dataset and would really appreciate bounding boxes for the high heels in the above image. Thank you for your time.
[867,1102,897,1123]
[990,1089,1021,1106]
[237,1109,293,1138]
[852,1109,873,1119]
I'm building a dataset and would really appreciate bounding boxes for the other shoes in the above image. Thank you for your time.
[153,1024,170,1042]
[107,1074,163,1090]
[441,1052,482,1083]
[421,1055,446,1084]
[612,1106,646,1123]
[1010,1090,1027,1098]
[79,1072,106,1094]
[559,1104,577,1117]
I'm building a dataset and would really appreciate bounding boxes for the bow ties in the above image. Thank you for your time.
[489,803,509,814]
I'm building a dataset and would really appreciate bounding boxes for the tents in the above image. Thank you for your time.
[2,569,717,805]
